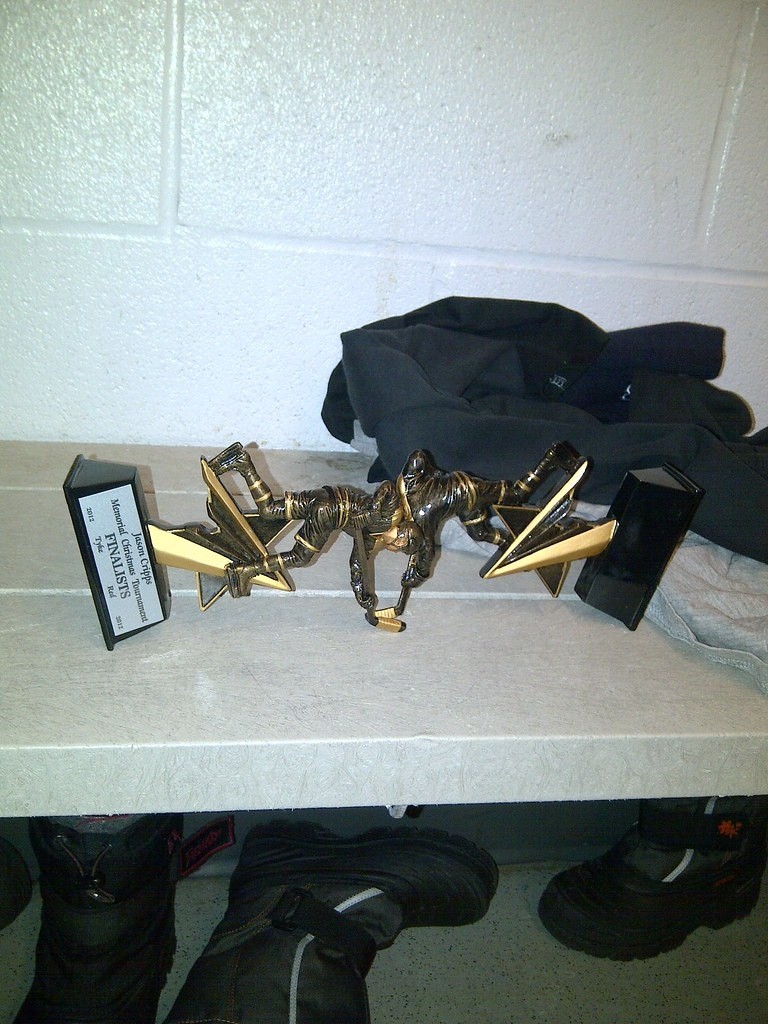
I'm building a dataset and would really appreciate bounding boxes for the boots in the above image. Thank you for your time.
[14,815,235,1024]
[165,818,500,1024]
[539,794,768,961]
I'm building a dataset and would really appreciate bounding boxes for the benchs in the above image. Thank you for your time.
[0,435,768,821]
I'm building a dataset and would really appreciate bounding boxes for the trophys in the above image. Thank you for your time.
[373,439,705,633]
[63,410,424,651]
